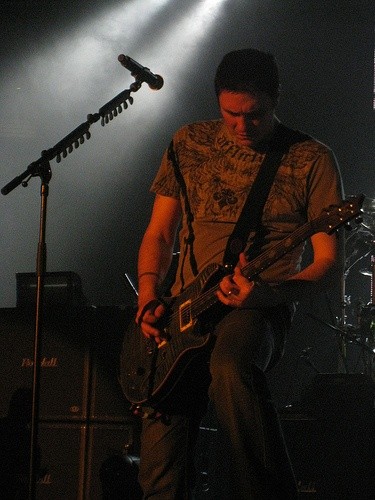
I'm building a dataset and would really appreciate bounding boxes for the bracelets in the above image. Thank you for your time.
[137,271,160,280]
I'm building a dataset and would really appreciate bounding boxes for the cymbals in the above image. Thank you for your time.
[359,266,375,276]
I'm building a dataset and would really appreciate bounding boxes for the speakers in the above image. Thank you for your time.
[0,306,144,500]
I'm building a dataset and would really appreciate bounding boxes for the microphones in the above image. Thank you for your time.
[118,54,164,91]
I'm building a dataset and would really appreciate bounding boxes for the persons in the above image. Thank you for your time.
[133,43,347,500]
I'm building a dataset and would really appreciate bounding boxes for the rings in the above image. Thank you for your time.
[226,291,232,297]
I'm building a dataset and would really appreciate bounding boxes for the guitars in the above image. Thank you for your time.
[118,191,367,408]
